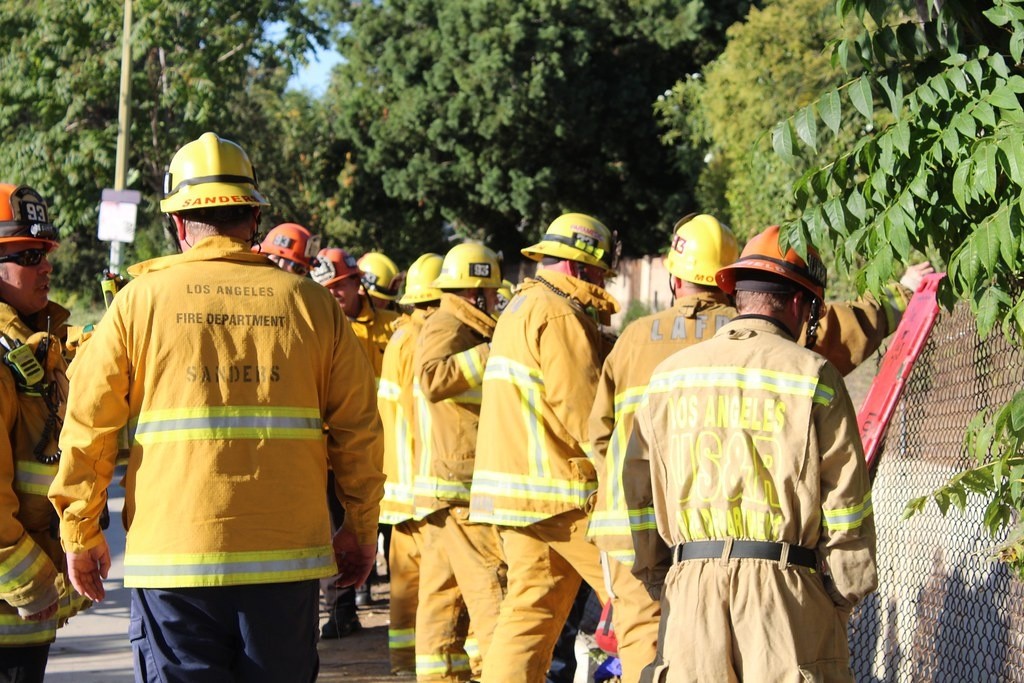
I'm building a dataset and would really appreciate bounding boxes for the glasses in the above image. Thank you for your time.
[283,258,305,274]
[0,248,47,267]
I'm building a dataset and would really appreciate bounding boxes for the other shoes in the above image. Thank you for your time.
[355,591,372,605]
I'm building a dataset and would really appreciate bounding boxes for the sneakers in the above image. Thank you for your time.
[322,610,360,638]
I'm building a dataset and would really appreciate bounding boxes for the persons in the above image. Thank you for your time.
[0,183,110,683]
[253,223,515,683]
[45,130,388,682]
[410,240,505,683]
[619,224,878,683]
[472,212,616,683]
[581,211,934,683]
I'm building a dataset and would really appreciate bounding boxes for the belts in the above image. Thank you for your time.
[669,540,817,570]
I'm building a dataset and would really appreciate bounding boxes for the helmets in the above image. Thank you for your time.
[398,252,444,304]
[715,225,828,320]
[251,223,319,271]
[311,247,364,287]
[159,132,272,213]
[0,183,60,252]
[427,242,511,290]
[520,212,618,277]
[664,213,739,287]
[356,252,401,300]
